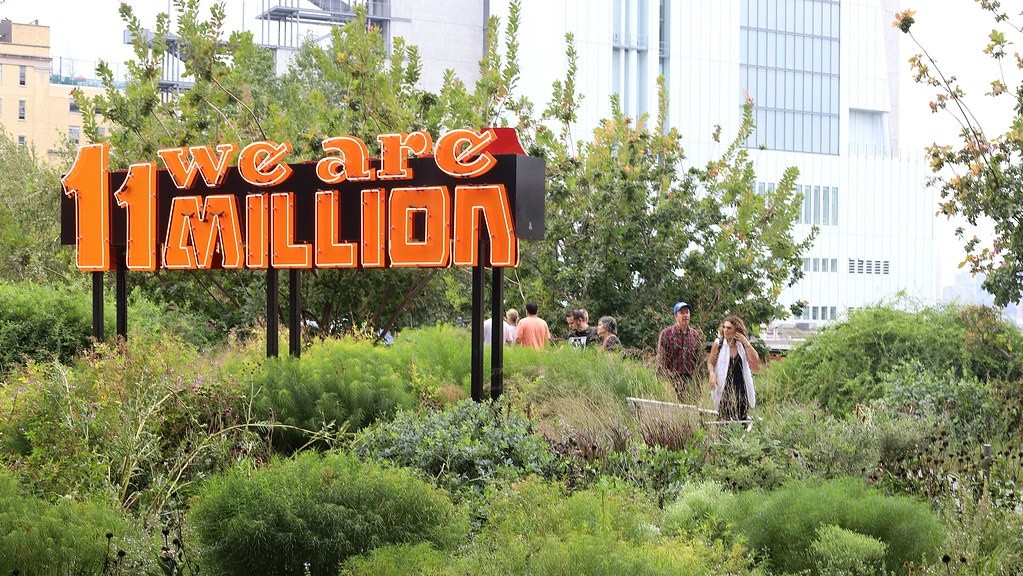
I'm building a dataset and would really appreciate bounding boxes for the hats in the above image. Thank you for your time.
[673,302,692,313]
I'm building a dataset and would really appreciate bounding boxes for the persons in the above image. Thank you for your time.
[566,309,621,352]
[707,315,760,441]
[656,302,705,399]
[484,303,551,349]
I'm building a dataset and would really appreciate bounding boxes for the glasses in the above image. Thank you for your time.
[722,325,731,329]
